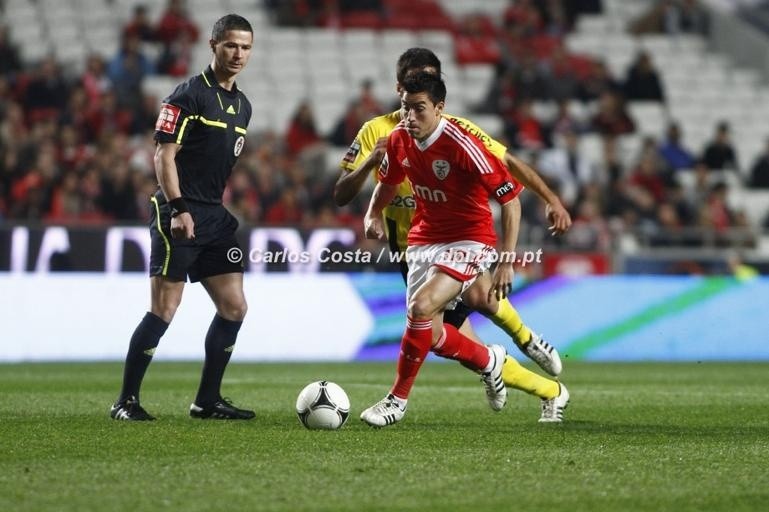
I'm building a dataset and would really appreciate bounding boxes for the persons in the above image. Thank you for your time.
[110,14,254,420]
[0,0,768,278]
[359,69,525,427]
[334,46,573,422]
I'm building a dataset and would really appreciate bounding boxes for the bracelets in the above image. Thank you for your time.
[166,197,190,218]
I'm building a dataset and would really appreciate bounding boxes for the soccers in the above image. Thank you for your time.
[296,380,350,430]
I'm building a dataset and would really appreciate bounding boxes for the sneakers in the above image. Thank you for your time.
[189,397,256,420]
[359,390,408,429]
[512,326,563,376]
[476,343,508,412]
[110,395,158,421]
[536,376,570,423]
